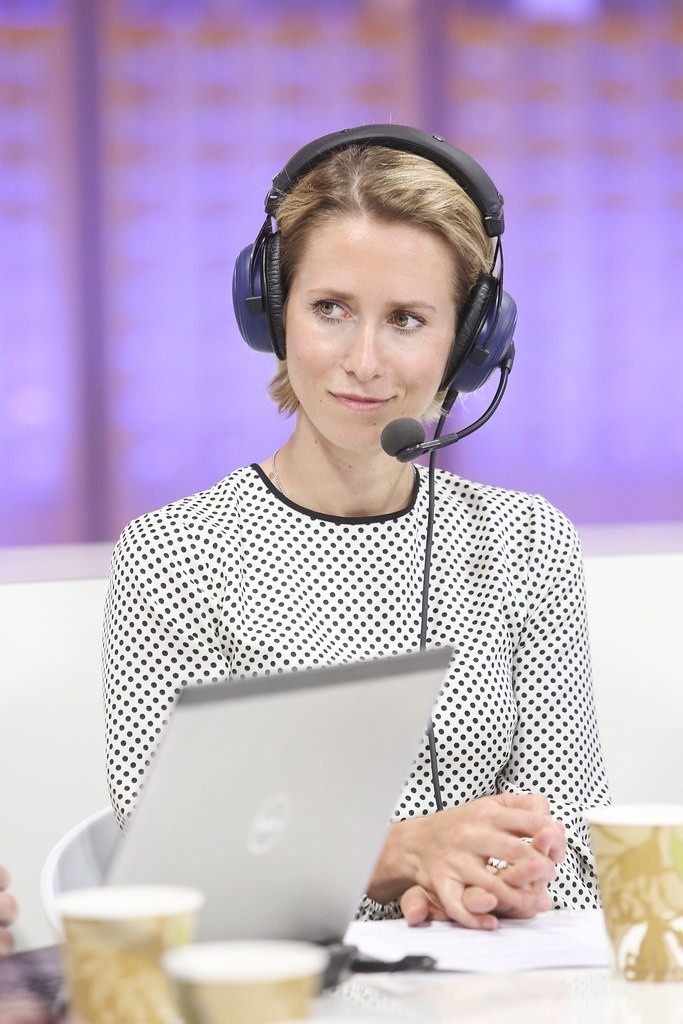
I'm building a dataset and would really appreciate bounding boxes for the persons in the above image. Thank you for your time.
[0,863,19,958]
[105,121,613,932]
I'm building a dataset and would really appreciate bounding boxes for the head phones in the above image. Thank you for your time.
[231,124,519,393]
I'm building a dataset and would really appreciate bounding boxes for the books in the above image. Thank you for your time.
[342,907,618,973]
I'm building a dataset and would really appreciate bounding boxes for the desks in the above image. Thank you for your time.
[0,908,683,1024]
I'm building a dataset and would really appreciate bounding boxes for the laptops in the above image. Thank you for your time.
[0,645,454,1024]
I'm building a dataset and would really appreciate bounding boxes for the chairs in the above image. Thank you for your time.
[38,805,119,939]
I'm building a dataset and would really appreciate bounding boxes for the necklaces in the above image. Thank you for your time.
[271,447,418,509]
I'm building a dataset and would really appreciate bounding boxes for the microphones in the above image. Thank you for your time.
[381,369,511,462]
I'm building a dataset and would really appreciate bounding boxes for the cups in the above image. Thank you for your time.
[583,804,683,985]
[160,935,329,1024]
[56,883,203,1024]
